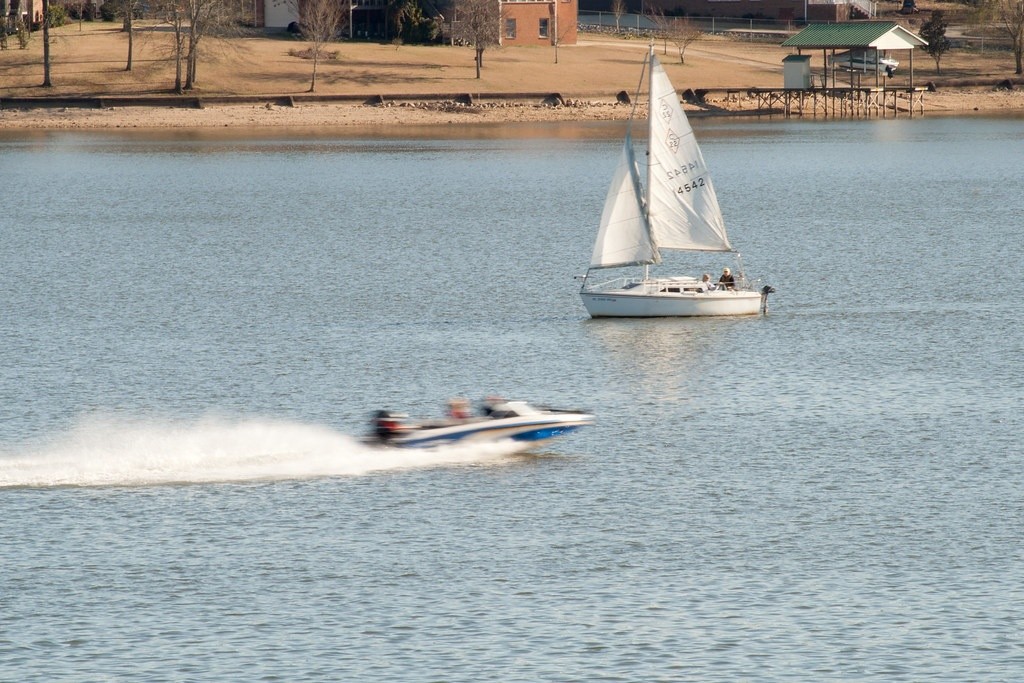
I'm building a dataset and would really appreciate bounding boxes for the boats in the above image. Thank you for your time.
[840,56,901,72]
[364,395,595,448]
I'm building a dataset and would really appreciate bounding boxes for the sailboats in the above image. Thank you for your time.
[578,33,764,317]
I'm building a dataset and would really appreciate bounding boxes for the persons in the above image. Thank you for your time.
[694,274,722,291]
[718,268,735,290]
